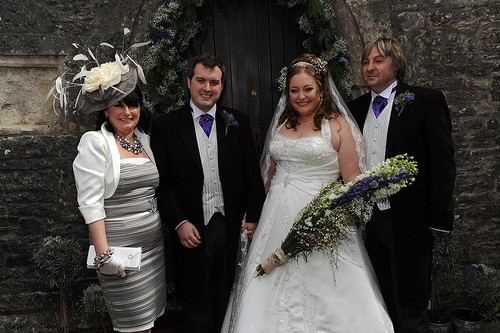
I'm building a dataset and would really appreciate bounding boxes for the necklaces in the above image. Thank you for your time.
[298,120,314,127]
[113,132,143,155]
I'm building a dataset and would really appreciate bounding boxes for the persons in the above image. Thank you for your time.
[345,36,456,333]
[46,27,168,333]
[220,53,395,333]
[150,51,267,333]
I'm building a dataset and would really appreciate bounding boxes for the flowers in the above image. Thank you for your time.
[464,263,500,328]
[394,90,414,116]
[255,152,419,288]
[44,27,152,117]
[222,110,239,137]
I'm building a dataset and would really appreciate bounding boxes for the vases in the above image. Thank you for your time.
[450,308,486,333]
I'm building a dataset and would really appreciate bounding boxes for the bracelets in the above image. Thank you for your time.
[93,247,114,269]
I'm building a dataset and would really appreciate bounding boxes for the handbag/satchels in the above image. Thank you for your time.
[86,245,142,270]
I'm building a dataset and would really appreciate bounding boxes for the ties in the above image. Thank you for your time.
[372,85,398,119]
[190,106,214,138]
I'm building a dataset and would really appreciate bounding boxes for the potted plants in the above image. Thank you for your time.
[427,228,471,333]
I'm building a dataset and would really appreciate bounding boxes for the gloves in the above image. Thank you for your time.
[98,254,126,278]
